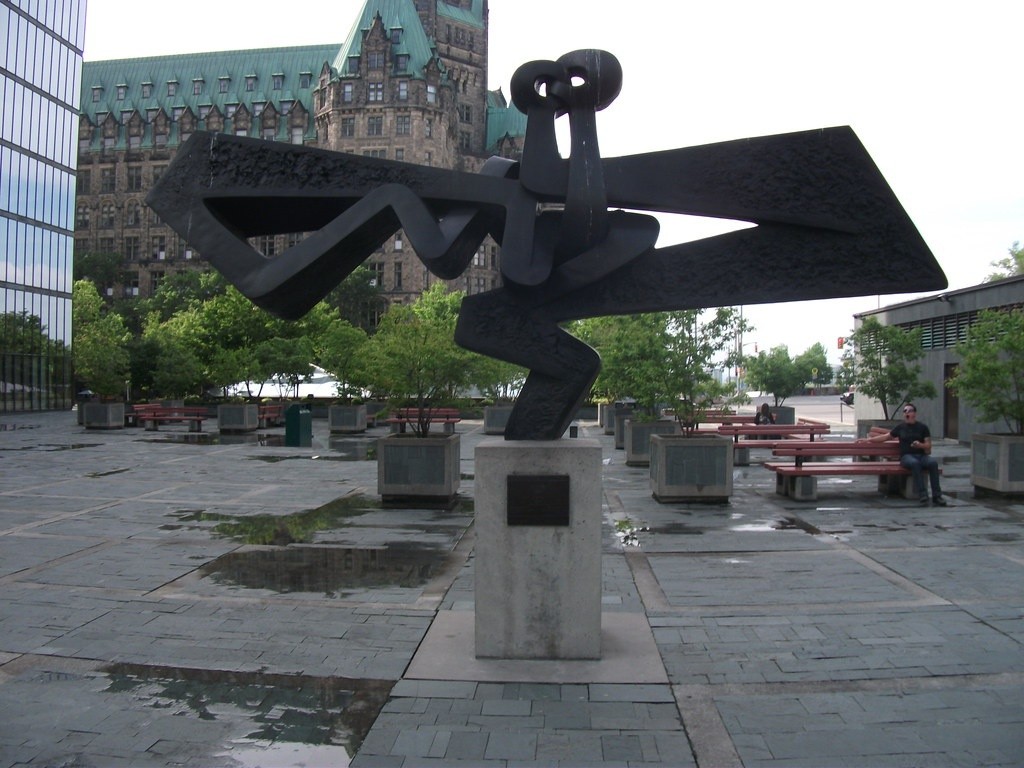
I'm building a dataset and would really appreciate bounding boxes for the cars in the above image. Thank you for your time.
[205,363,372,399]
[840,391,854,405]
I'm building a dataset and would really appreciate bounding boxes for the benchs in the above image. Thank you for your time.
[765,441,942,503]
[852,427,900,462]
[124,404,166,426]
[140,406,208,432]
[257,405,283,428]
[781,419,826,441]
[386,409,462,433]
[681,415,756,440]
[718,424,831,467]
[665,410,736,428]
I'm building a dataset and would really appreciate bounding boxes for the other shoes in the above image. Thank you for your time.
[919,496,929,506]
[932,496,946,505]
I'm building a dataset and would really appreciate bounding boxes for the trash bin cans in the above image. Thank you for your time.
[285,403,312,447]
[77,390,94,424]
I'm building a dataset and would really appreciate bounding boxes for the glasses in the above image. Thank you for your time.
[903,409,915,413]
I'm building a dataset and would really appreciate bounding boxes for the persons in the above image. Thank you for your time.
[855,404,946,506]
[754,403,777,440]
